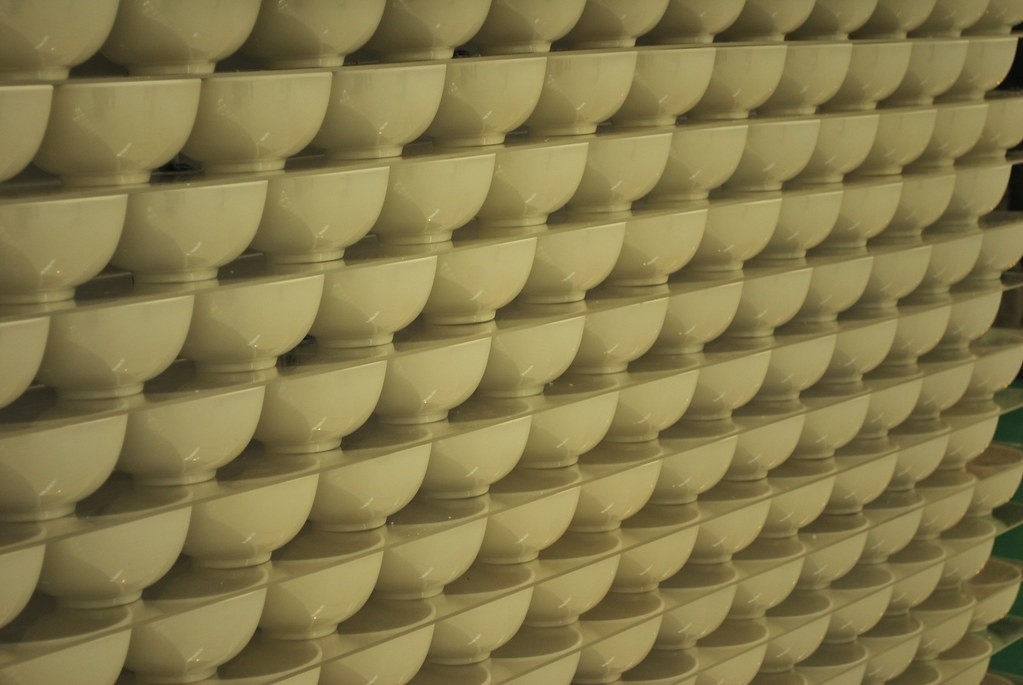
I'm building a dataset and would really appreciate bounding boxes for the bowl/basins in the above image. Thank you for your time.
[0,0,1023,685]
